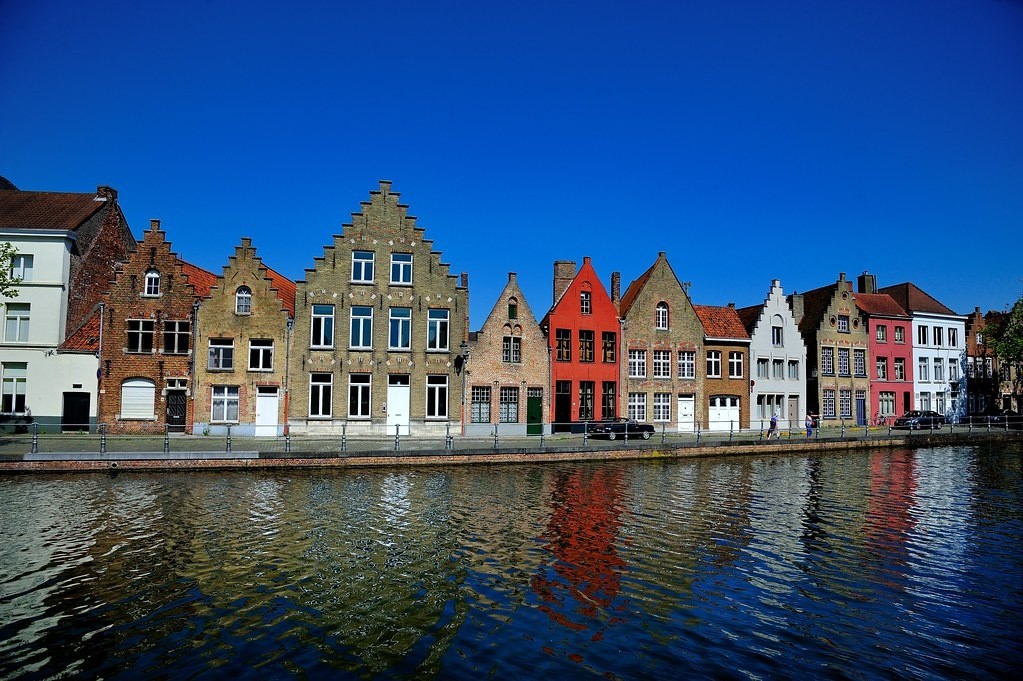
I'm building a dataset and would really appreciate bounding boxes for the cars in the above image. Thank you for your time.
[589,417,655,442]
[894,410,946,430]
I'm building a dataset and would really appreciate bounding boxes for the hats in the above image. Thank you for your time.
[808,411,813,414]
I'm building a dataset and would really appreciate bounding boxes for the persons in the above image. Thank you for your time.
[767,412,780,439]
[805,410,821,439]
[873,412,886,425]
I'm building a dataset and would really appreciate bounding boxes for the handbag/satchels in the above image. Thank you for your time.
[812,422,814,425]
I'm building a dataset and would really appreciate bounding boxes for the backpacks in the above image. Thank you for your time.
[770,418,776,429]
